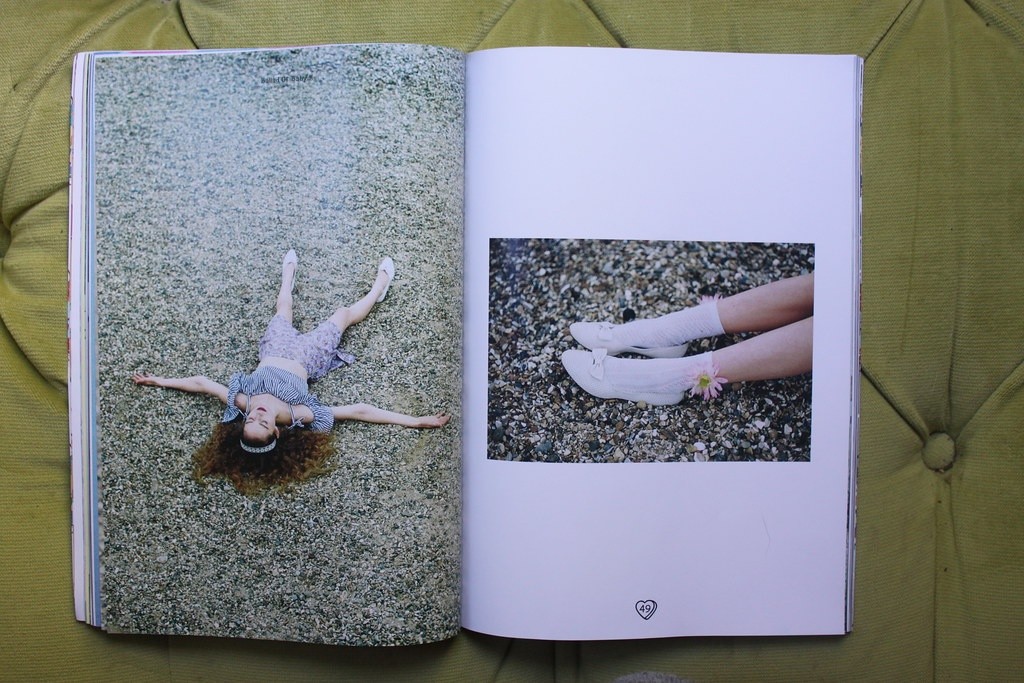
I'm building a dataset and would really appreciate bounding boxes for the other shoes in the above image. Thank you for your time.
[282,249,297,292]
[560,349,686,405]
[373,257,395,302]
[569,320,689,358]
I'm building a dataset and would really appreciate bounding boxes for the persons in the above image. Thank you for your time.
[130,246,451,493]
[561,269,812,407]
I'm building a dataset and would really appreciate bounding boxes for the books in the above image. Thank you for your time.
[67,42,863,647]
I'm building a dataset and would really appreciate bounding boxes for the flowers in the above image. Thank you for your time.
[687,360,728,400]
[698,292,723,303]
